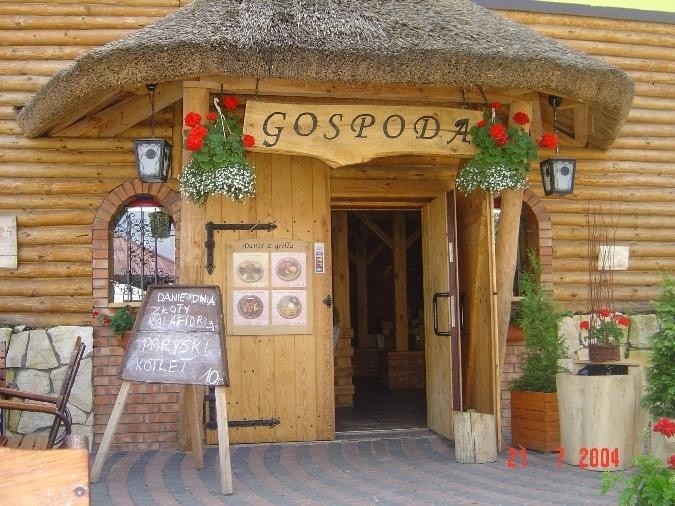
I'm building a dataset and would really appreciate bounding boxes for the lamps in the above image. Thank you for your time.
[540,96,576,196]
[133,82,172,184]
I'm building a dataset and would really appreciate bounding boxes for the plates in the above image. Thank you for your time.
[238,258,303,318]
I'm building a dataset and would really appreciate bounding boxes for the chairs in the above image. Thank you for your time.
[0,342,7,438]
[0,337,86,449]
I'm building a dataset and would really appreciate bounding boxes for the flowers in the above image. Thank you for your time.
[652,418,675,475]
[477,102,556,152]
[185,97,254,151]
[581,308,629,333]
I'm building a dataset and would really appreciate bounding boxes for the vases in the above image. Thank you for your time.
[590,345,621,362]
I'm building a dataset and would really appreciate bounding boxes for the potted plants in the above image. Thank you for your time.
[507,250,562,455]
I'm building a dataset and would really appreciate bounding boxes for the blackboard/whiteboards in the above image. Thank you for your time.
[118,285,230,385]
